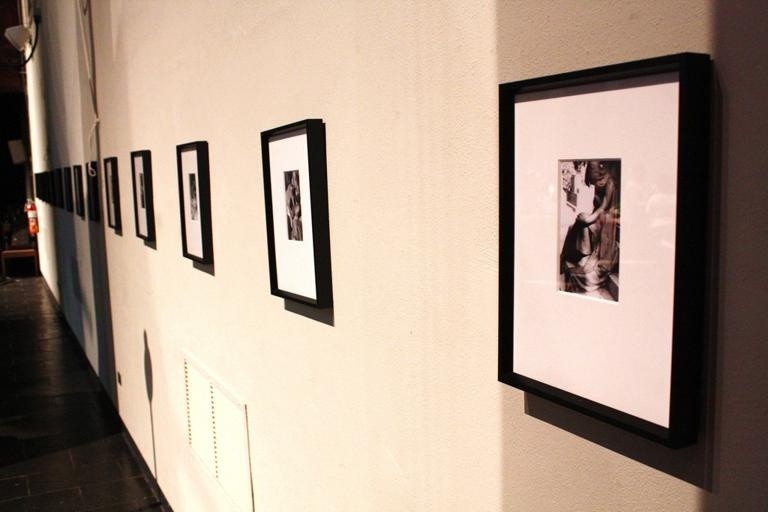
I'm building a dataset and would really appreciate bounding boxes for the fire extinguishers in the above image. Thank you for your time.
[24,198,39,236]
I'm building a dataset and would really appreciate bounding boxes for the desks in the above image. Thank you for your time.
[1,247,39,278]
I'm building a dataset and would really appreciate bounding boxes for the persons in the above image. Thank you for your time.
[560,160,618,291]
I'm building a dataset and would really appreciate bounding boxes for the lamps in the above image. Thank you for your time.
[3,4,33,53]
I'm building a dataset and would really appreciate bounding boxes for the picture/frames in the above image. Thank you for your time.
[103,157,121,230]
[261,120,333,310]
[130,150,155,243]
[35,161,100,223]
[177,141,212,265]
[497,52,709,454]
